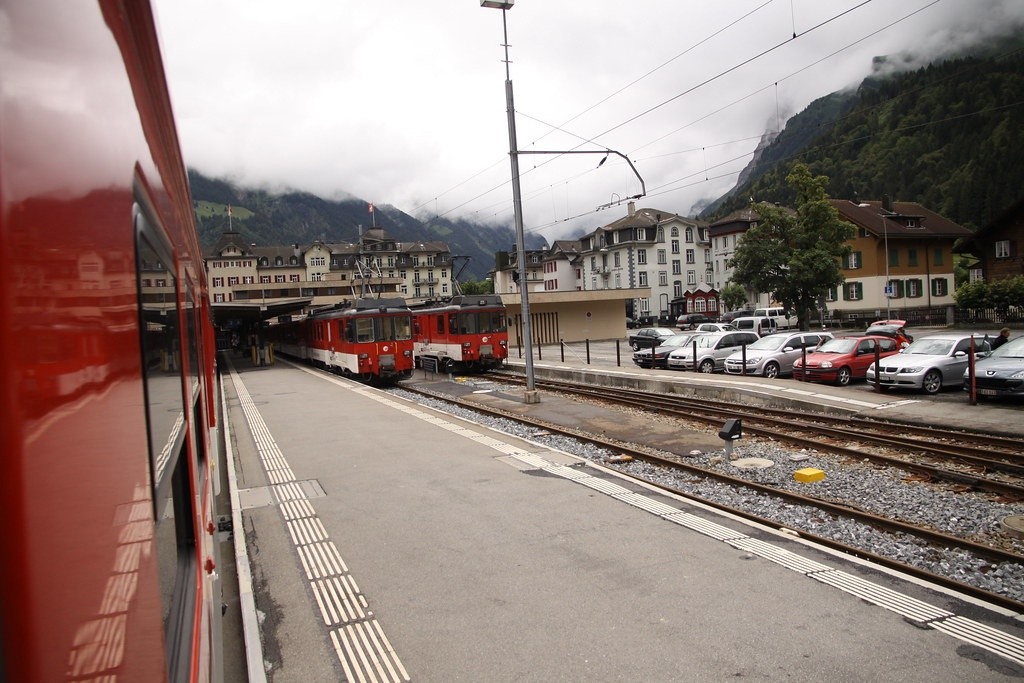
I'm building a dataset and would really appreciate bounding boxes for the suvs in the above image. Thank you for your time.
[675,313,716,331]
[721,310,756,324]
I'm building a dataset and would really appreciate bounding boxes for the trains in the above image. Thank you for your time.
[0,0,221,683]
[270,297,416,387]
[408,293,508,376]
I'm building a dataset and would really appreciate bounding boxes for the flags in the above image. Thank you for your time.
[369,204,373,213]
[228,207,232,216]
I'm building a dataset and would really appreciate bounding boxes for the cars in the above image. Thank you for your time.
[963,335,1024,407]
[625,316,642,329]
[791,320,913,386]
[723,330,835,380]
[667,330,760,374]
[628,328,677,353]
[632,333,701,371]
[865,334,998,395]
[695,322,740,332]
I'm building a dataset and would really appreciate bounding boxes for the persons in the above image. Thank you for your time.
[993,328,1010,350]
[231,336,239,354]
[897,328,906,337]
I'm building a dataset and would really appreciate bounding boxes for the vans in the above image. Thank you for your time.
[753,307,800,330]
[729,316,778,338]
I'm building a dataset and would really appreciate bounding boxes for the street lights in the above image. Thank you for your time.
[479,0,540,404]
